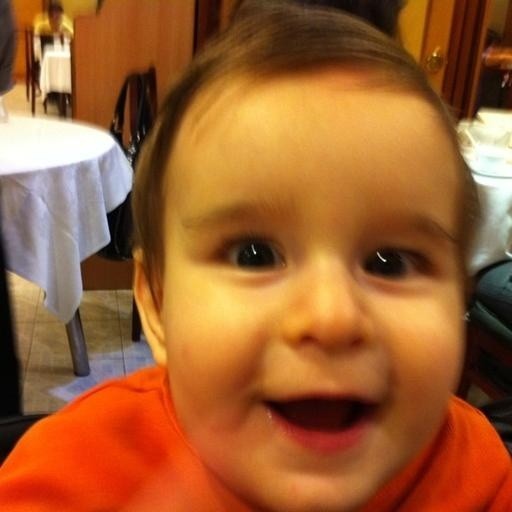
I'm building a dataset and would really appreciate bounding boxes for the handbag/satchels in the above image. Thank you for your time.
[96,74,149,262]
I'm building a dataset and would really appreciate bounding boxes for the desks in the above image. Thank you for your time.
[0,114,134,377]
[467,176,512,279]
[39,46,71,119]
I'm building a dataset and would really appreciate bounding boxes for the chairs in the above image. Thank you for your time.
[24,26,40,116]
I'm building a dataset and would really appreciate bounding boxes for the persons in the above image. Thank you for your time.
[33,0,73,34]
[2,1,511,510]
[31,2,73,102]
